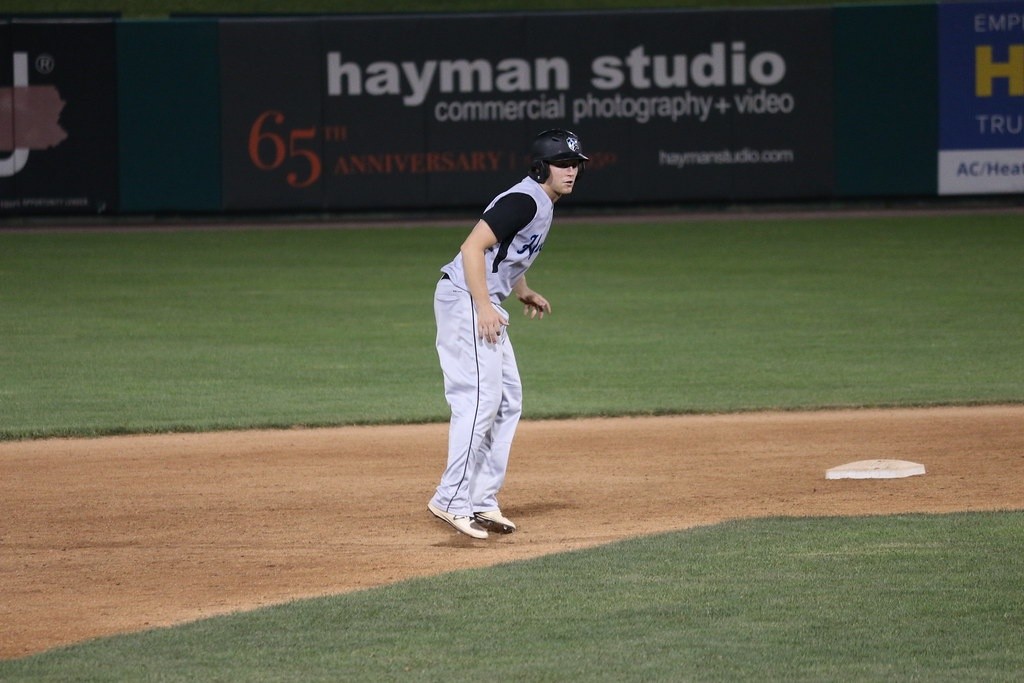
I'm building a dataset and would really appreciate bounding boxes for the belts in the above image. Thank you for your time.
[442,273,450,279]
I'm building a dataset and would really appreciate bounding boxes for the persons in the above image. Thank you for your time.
[429,126,589,539]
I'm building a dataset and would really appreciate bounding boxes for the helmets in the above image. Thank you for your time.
[528,128,589,184]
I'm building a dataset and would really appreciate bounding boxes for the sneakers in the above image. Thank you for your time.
[472,506,516,533]
[427,503,489,539]
